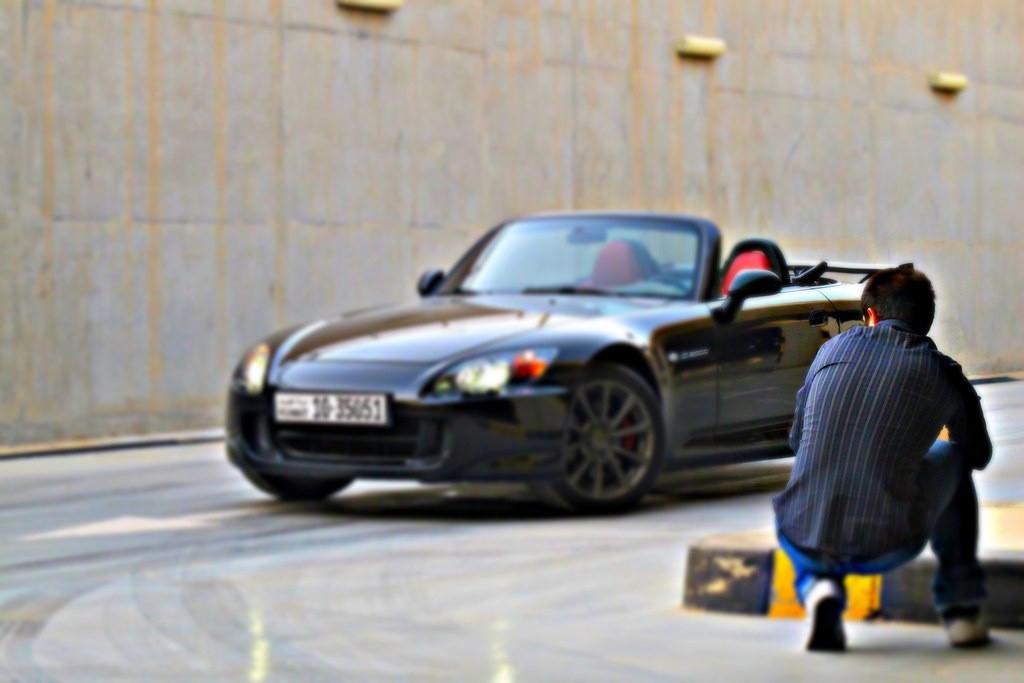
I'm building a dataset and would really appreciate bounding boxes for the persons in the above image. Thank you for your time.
[773,266,992,651]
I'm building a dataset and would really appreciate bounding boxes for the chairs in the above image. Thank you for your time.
[717,238,790,297]
[595,238,659,287]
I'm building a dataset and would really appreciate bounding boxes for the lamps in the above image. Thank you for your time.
[676,35,725,58]
[932,71,968,90]
[336,0,404,12]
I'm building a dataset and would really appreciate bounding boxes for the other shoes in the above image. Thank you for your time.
[803,579,846,651]
[939,601,989,645]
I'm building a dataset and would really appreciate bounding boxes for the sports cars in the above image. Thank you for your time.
[222,207,896,520]
